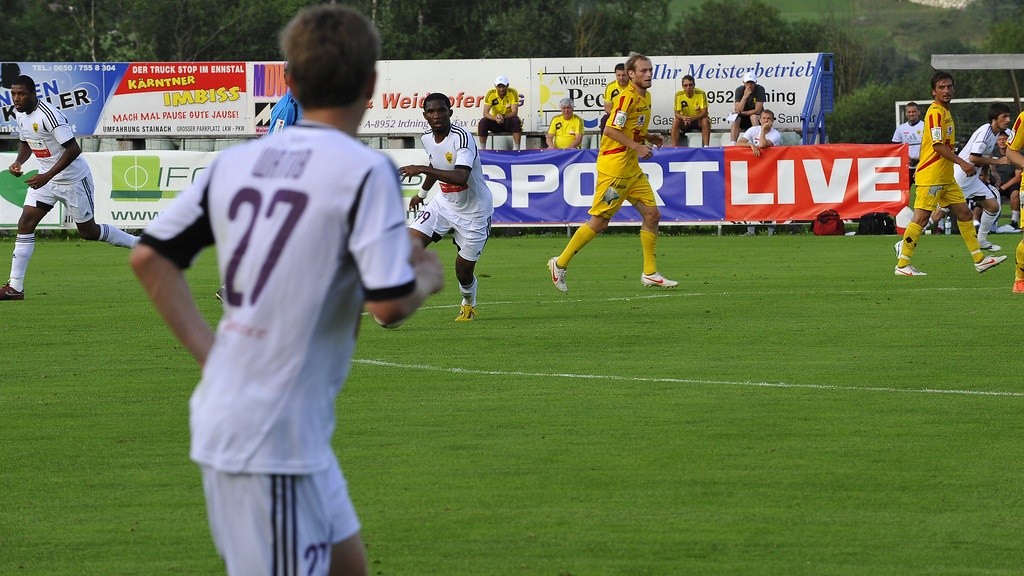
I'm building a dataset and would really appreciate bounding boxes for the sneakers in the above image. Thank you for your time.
[895,264,927,276]
[0,283,24,301]
[547,257,568,292]
[974,255,1007,273]
[454,299,477,321]
[894,241,903,260]
[980,243,1002,252]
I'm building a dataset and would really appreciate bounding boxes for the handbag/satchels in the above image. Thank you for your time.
[641,272,679,289]
[856,214,897,235]
[813,210,846,235]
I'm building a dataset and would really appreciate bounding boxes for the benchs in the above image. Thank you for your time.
[0,128,825,152]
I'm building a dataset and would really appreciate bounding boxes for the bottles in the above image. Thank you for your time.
[945,216,951,235]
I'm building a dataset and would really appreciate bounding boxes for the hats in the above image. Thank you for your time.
[494,75,508,87]
[743,72,757,83]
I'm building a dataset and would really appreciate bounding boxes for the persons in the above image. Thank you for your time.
[894,102,1011,261]
[894,71,1007,276]
[892,102,924,184]
[671,75,711,147]
[966,164,1001,234]
[397,93,494,320]
[547,53,679,291]
[731,72,766,145]
[989,131,1020,229]
[1005,110,1024,294]
[130,6,445,576]
[478,76,522,150]
[546,98,584,149]
[0,75,141,302]
[735,109,781,156]
[601,64,632,134]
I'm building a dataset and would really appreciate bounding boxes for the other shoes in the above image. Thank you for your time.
[1010,220,1018,229]
[974,225,981,232]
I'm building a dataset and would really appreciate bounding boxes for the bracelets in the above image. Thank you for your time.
[418,188,428,198]
